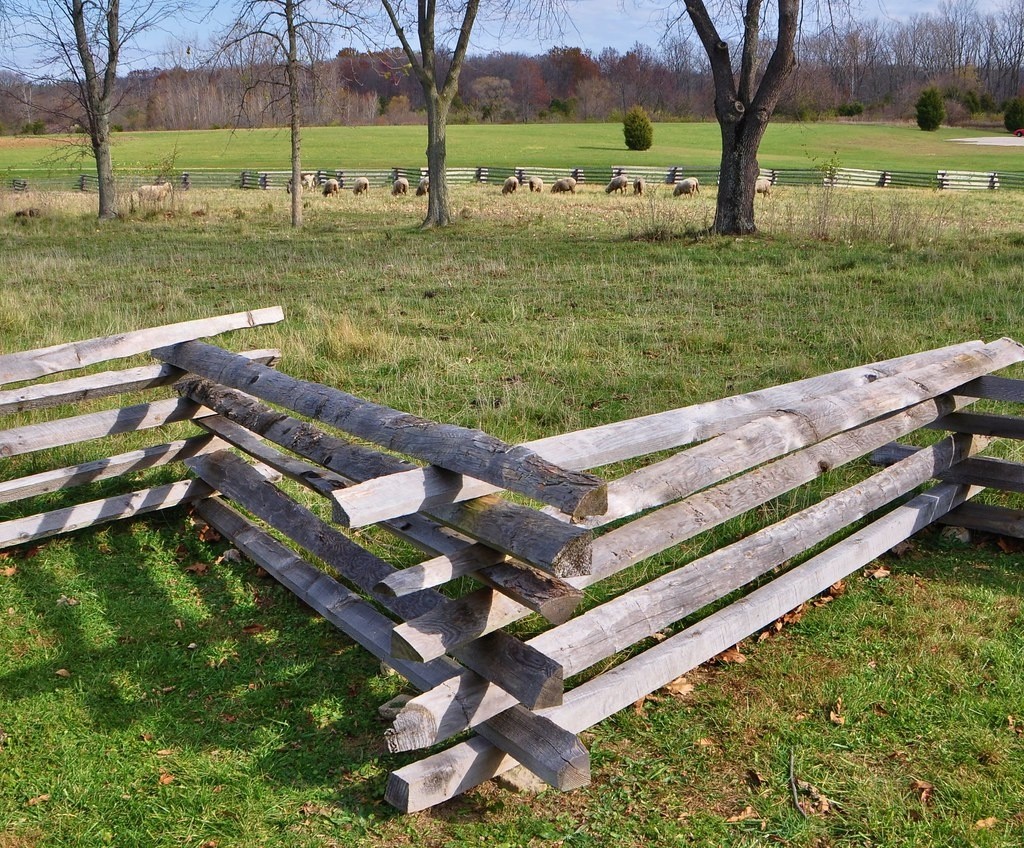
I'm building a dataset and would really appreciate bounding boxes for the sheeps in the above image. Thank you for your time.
[390,178,409,196]
[502,177,518,195]
[605,175,628,195]
[633,175,645,196]
[550,177,577,195]
[322,179,339,197]
[300,173,317,195]
[673,177,701,198]
[352,177,369,198]
[415,177,429,196]
[755,179,771,197]
[137,182,173,210]
[529,176,544,193]
